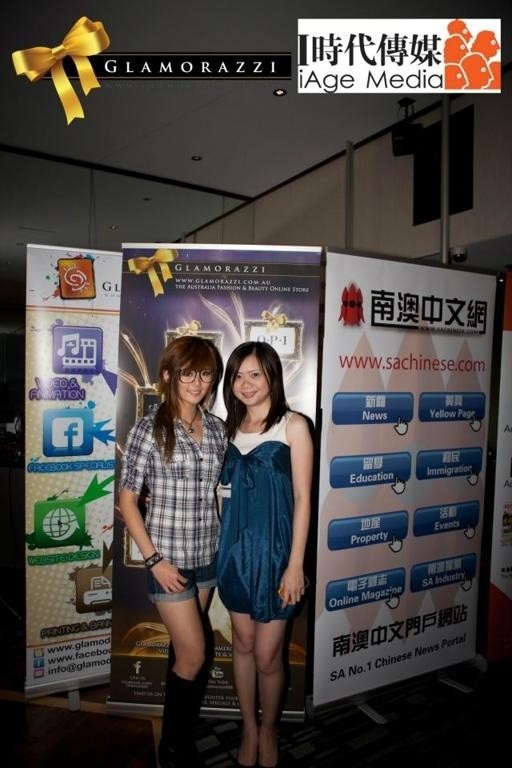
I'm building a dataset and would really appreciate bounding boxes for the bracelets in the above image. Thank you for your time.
[144,552,163,573]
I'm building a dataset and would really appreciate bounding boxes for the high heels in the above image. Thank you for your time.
[235,723,284,768]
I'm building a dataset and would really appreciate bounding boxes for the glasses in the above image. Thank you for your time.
[177,368,214,383]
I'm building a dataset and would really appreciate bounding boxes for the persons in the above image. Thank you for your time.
[213,339,314,768]
[119,333,230,767]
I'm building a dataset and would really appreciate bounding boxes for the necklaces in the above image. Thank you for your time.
[183,409,200,434]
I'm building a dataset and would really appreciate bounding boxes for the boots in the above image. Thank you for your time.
[156,660,206,766]
